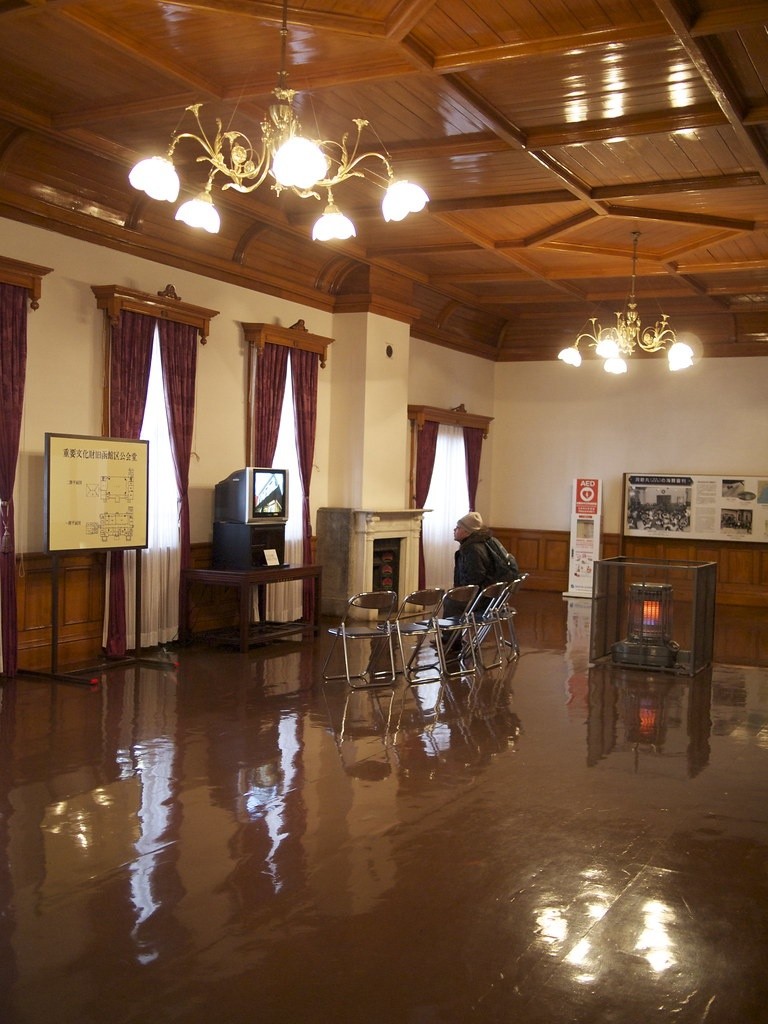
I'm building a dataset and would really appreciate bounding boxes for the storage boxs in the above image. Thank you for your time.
[585,667,712,782]
[585,554,719,678]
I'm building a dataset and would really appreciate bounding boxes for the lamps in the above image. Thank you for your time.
[126,0,433,243]
[609,582,680,668]
[624,671,672,745]
[555,229,696,377]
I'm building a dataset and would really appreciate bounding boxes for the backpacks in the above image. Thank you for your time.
[481,537,518,588]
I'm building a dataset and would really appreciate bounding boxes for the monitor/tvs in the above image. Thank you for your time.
[213,467,289,525]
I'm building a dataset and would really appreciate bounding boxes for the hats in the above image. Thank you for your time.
[457,512,482,532]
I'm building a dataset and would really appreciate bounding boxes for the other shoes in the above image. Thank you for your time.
[430,639,436,643]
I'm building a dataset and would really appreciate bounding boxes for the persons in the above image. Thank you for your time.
[430,512,493,658]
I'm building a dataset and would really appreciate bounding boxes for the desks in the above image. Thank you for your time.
[183,562,323,653]
[172,651,319,726]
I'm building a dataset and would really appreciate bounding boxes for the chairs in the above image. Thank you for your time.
[316,658,523,781]
[467,578,522,659]
[411,585,480,679]
[478,572,533,653]
[446,581,506,671]
[371,586,446,683]
[320,590,397,689]
